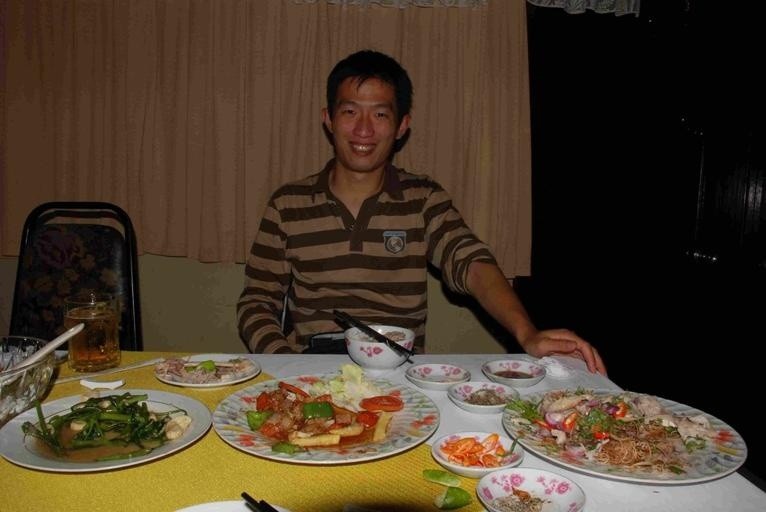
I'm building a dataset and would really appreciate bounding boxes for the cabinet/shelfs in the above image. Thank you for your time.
[513,1,766,491]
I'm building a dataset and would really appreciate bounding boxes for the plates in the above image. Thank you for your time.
[1,388,210,471]
[408,361,467,387]
[450,382,519,413]
[157,353,260,389]
[474,470,588,510]
[211,375,439,463]
[431,435,521,476]
[482,361,546,387]
[505,388,748,487]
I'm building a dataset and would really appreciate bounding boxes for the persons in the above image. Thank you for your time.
[234,49,608,378]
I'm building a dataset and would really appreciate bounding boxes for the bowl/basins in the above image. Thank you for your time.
[347,325,415,374]
[2,335,55,419]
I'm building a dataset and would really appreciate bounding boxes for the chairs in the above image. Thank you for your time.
[4,201,143,350]
[279,287,294,337]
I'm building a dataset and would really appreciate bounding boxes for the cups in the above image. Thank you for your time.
[63,294,121,371]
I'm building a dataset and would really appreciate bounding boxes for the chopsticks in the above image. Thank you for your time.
[329,306,413,356]
[53,358,164,385]
[240,490,281,512]
